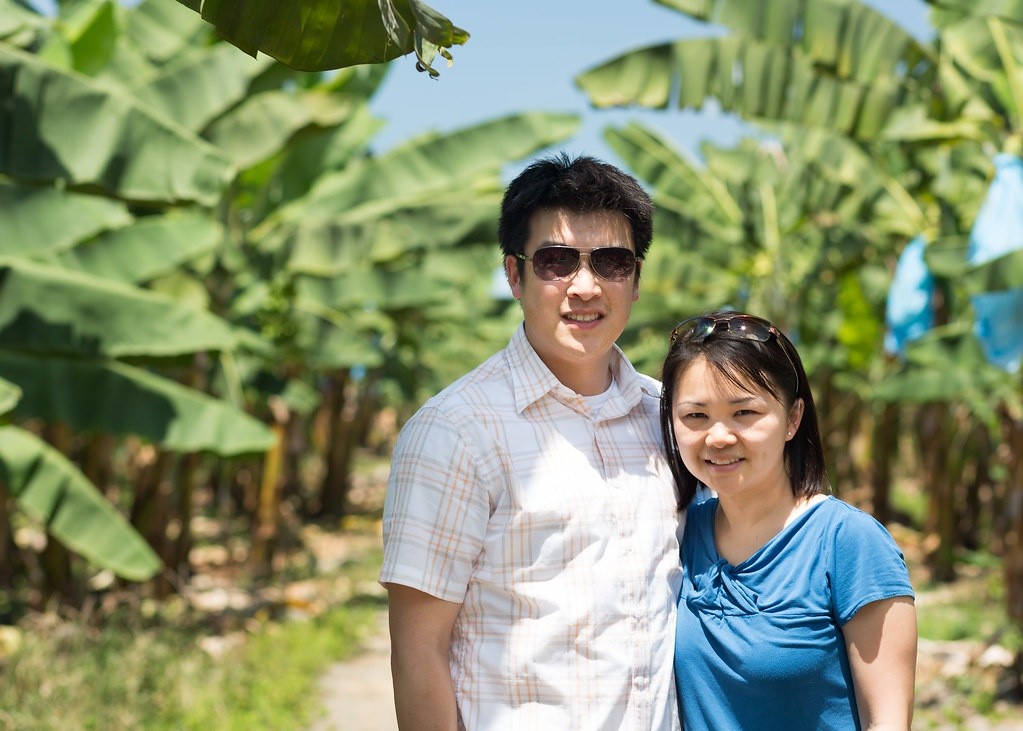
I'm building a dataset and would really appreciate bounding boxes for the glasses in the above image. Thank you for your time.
[507,246,640,284]
[670,316,799,396]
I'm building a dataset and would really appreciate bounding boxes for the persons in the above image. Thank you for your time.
[378,158,717,731]
[660,309,918,731]
